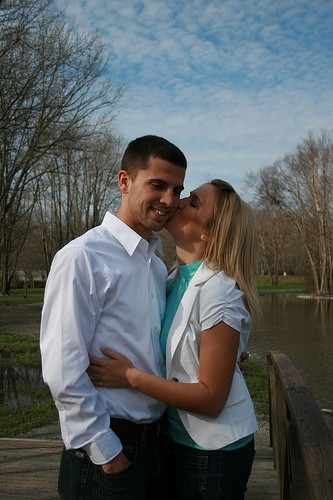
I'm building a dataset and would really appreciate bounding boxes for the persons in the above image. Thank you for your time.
[88,179,264,498]
[40,133,249,499]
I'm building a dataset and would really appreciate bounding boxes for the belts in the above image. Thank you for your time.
[107,413,171,444]
